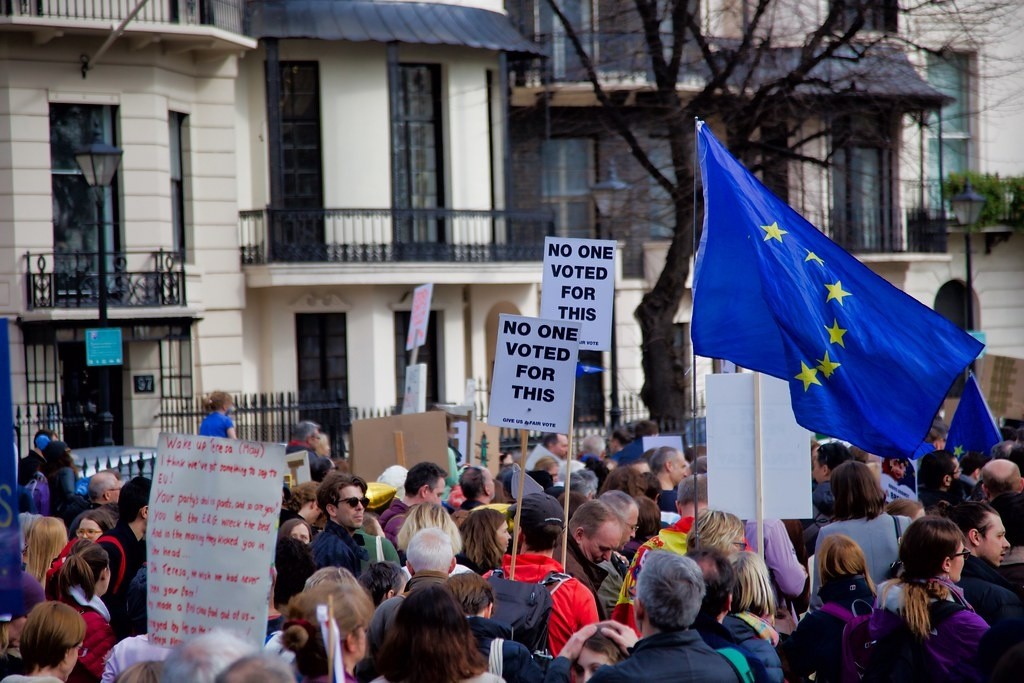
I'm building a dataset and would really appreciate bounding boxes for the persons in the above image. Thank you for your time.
[0,388,1024,683]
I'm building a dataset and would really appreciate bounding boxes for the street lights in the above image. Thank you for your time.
[67,123,122,445]
[947,172,986,378]
[593,158,632,436]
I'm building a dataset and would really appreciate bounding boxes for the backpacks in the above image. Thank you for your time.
[486,569,571,671]
[862,599,968,683]
[24,470,53,516]
[818,595,884,683]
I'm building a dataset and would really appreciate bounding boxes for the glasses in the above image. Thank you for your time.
[76,528,102,538]
[76,645,88,657]
[337,497,369,507]
[732,542,747,550]
[948,547,971,560]
[624,522,640,532]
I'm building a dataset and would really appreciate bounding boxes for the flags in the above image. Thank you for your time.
[690,120,1001,468]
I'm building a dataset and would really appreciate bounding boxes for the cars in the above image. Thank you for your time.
[62,444,162,487]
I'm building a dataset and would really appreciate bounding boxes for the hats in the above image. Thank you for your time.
[36,434,68,465]
[507,493,567,532]
